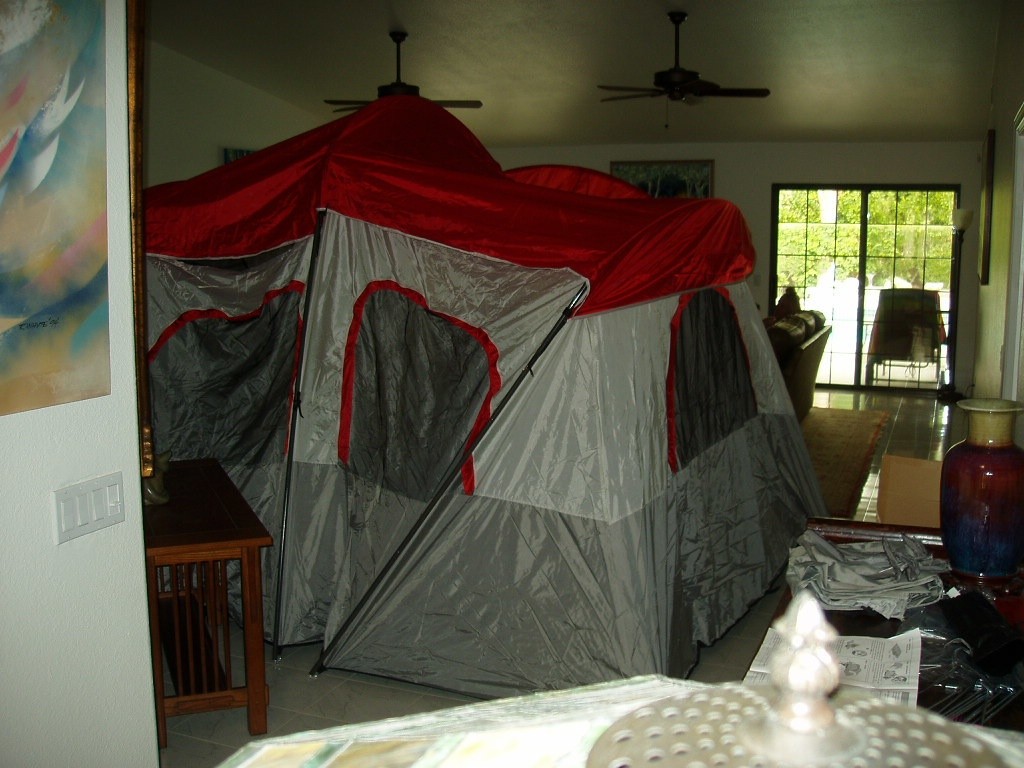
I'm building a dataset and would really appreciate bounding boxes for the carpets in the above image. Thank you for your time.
[801,407,892,519]
[878,453,943,527]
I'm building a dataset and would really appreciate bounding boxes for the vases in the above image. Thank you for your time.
[941,398,1024,577]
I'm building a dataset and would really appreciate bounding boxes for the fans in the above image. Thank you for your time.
[597,12,771,107]
[324,30,483,114]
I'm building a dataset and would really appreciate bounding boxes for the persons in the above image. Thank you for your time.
[757,287,800,329]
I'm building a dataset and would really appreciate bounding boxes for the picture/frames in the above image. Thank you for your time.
[610,158,715,199]
[977,129,996,286]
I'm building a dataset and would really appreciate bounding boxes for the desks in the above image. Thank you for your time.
[138,458,275,750]
[742,516,1024,732]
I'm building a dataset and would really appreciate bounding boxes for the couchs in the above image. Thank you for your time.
[766,310,832,423]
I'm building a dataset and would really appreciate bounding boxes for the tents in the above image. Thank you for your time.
[139,95,826,690]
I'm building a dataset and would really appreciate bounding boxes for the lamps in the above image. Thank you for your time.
[952,207,973,238]
